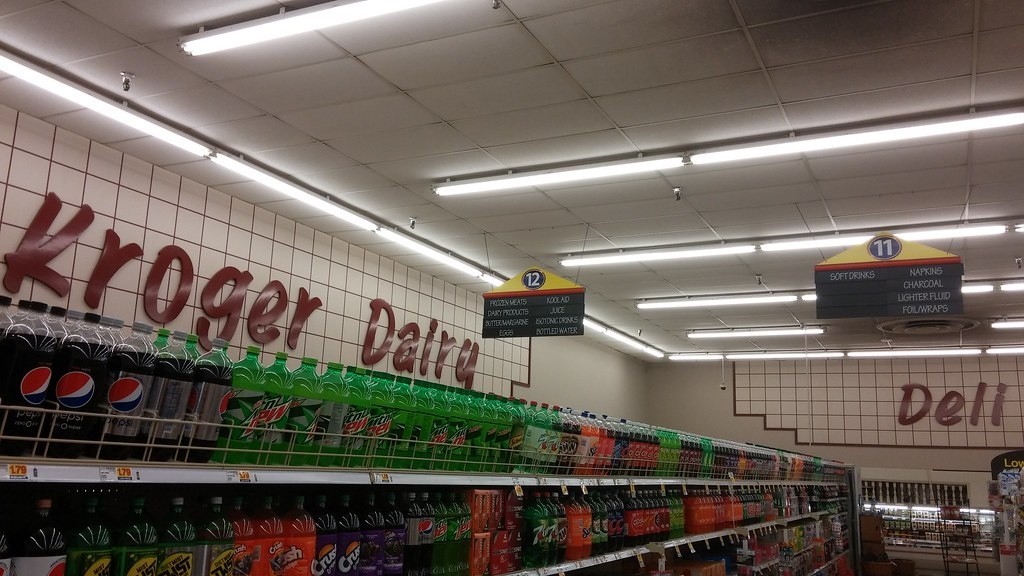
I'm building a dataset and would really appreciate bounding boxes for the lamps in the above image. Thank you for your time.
[0,0,1024,364]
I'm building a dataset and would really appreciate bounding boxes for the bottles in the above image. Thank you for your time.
[0,295,849,576]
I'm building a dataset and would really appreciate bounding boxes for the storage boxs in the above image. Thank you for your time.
[882,507,982,544]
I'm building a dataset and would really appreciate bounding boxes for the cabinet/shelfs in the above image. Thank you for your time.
[864,501,995,551]
[0,404,854,576]
